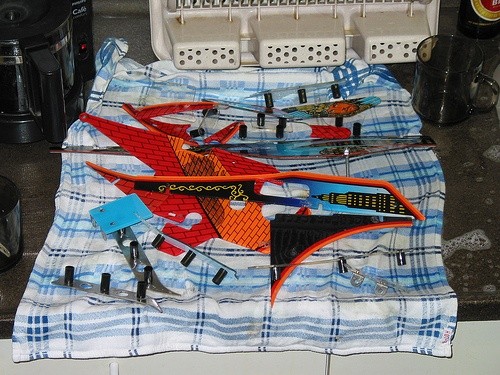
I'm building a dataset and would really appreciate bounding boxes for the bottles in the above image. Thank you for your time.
[455,0,500,39]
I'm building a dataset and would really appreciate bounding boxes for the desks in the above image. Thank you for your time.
[2,2,498,374]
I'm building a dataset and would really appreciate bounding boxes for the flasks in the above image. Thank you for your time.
[0,0,87,146]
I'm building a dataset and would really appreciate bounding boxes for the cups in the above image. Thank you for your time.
[0,174,25,275]
[411,34,500,127]
